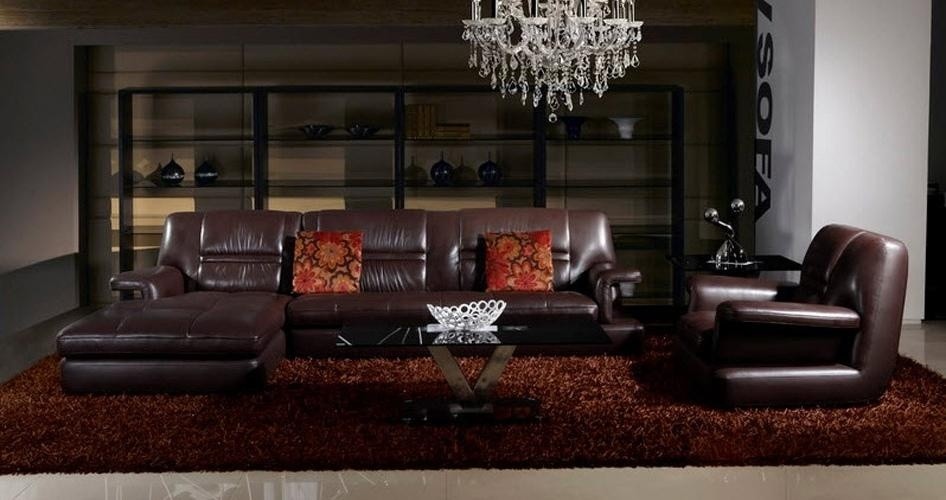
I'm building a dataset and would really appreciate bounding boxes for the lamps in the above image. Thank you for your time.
[461,0,644,122]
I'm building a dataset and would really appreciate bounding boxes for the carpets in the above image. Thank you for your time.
[0,346,704,471]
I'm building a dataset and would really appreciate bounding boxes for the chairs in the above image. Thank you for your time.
[664,223,909,408]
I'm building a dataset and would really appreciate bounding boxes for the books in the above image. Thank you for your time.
[405,102,476,140]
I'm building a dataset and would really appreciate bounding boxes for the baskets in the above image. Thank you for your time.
[425,300,506,329]
[433,331,502,345]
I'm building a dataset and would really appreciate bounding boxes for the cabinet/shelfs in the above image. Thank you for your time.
[112,85,686,322]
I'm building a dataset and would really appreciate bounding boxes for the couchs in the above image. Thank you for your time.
[56,206,646,397]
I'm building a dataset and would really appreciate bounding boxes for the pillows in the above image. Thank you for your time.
[290,231,362,295]
[481,231,555,293]
